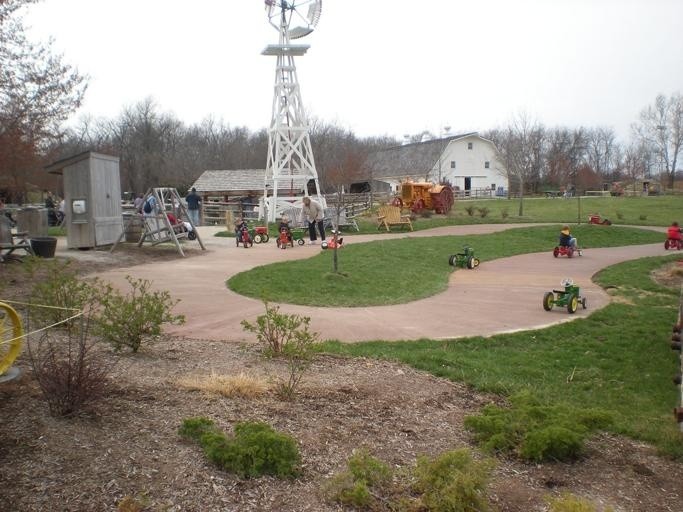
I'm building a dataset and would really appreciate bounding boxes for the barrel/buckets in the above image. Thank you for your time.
[30,237,57,258]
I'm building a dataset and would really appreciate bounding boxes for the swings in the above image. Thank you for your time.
[142,190,189,247]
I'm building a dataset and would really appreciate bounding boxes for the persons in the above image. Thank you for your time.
[667,221,682,242]
[132,192,144,216]
[141,190,161,235]
[184,187,202,229]
[54,193,65,223]
[276,214,292,245]
[232,217,253,243]
[299,195,328,246]
[557,225,577,248]
[42,188,58,226]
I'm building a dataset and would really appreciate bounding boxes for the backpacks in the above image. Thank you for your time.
[144,198,153,213]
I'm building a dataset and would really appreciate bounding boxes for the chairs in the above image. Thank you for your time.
[48,207,64,225]
[376,206,414,233]
[280,205,321,235]
[326,206,359,233]
[0,209,36,264]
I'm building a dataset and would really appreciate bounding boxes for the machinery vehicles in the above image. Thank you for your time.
[392,176,454,217]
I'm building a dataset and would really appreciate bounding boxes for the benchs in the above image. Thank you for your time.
[140,213,188,246]
